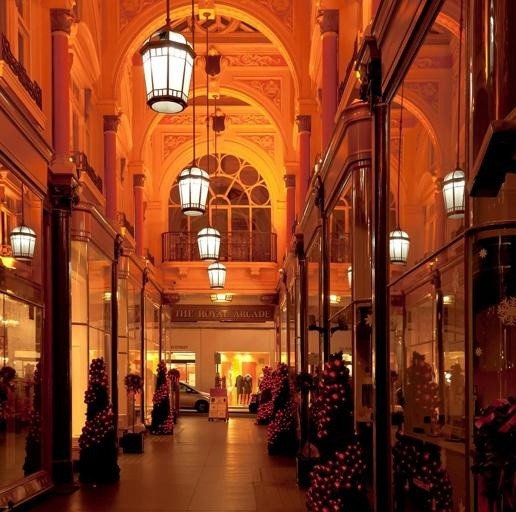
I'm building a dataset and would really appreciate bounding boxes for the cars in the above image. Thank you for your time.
[170,382,211,413]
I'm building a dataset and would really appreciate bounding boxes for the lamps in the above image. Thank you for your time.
[390,78,410,267]
[442,1,467,219]
[137,0,227,289]
[10,178,37,261]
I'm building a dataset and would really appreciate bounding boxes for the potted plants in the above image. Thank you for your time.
[122,373,144,453]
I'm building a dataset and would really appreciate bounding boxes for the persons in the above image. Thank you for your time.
[235,373,243,405]
[243,374,252,404]
